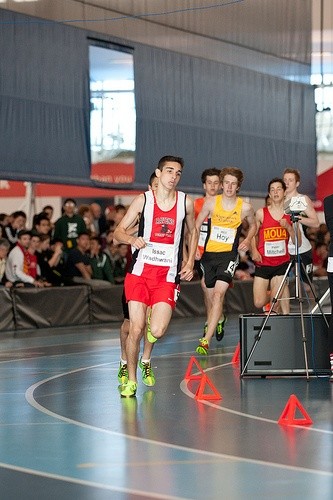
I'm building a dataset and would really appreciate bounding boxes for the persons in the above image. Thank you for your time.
[118,172,188,386]
[185,166,258,355]
[278,167,320,315]
[249,177,303,321]
[111,156,198,398]
[191,166,223,336]
[0,199,333,293]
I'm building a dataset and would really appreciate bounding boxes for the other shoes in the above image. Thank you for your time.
[262,303,271,315]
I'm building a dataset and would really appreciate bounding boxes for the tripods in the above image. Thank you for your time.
[240,216,331,380]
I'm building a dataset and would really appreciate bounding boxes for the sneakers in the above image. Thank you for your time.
[196,340,209,356]
[117,361,129,384]
[137,355,156,387]
[121,377,137,397]
[203,322,209,336]
[216,314,227,341]
[147,308,158,342]
